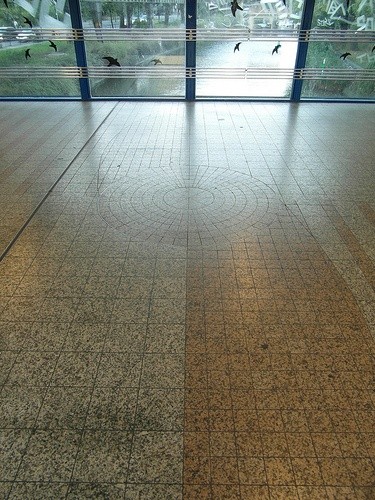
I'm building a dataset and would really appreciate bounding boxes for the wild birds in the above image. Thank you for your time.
[346,0,350,8]
[4,0,8,8]
[340,52,351,60]
[23,17,32,28]
[283,0,286,7]
[25,49,31,60]
[234,42,242,53]
[272,45,281,55]
[48,40,57,51]
[372,45,375,52]
[151,59,162,65]
[231,0,243,18]
[102,56,120,67]
